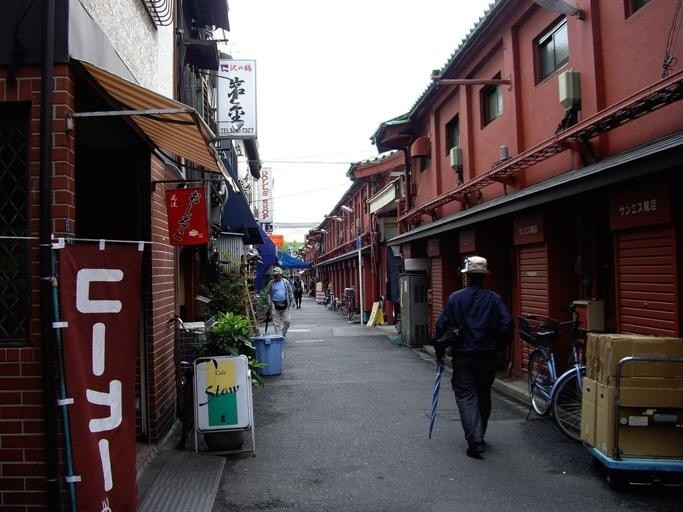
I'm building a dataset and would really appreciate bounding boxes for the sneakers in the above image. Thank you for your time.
[467,440,486,456]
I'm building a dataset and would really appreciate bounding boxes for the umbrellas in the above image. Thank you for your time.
[265,305,272,333]
[429,359,445,439]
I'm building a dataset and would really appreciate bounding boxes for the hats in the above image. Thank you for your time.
[461,256,489,274]
[274,267,281,275]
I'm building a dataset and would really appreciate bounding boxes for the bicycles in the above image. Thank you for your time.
[322,282,354,319]
[515,302,588,444]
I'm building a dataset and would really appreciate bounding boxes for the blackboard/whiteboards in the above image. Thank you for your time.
[194,355,252,432]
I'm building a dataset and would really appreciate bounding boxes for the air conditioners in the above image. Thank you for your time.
[178,320,206,368]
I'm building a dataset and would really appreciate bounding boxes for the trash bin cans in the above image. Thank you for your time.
[253,333,286,376]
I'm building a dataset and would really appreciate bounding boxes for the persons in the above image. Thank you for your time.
[287,270,306,309]
[433,255,515,457]
[267,267,293,339]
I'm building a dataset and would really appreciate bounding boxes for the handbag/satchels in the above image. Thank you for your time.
[273,300,287,310]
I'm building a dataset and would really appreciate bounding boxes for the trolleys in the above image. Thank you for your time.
[583,355,682,489]
